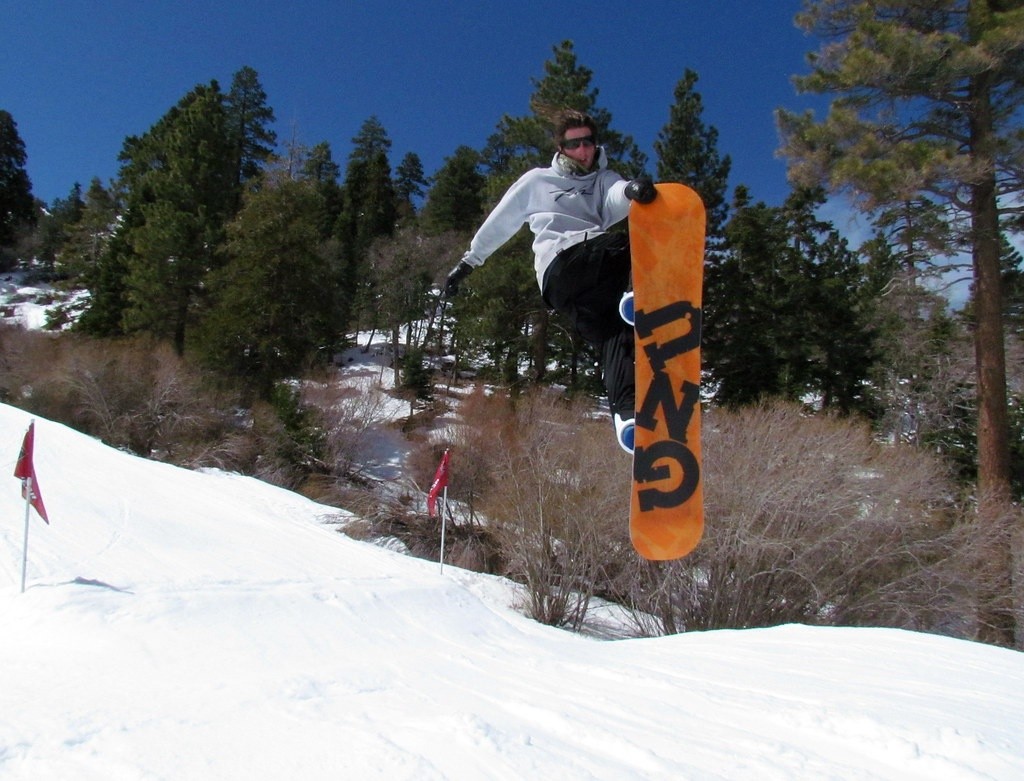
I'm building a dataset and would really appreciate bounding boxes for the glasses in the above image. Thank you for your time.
[562,135,596,150]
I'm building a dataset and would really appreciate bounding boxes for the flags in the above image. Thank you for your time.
[428,451,448,518]
[14,424,49,524]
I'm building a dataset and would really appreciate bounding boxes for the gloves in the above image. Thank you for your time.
[625,178,657,205]
[443,259,475,299]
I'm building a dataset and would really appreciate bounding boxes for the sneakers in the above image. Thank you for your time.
[619,286,634,327]
[613,410,635,456]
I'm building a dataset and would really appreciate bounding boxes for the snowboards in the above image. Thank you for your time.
[622,181,707,560]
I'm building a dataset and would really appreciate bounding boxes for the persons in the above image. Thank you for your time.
[445,110,658,455]
